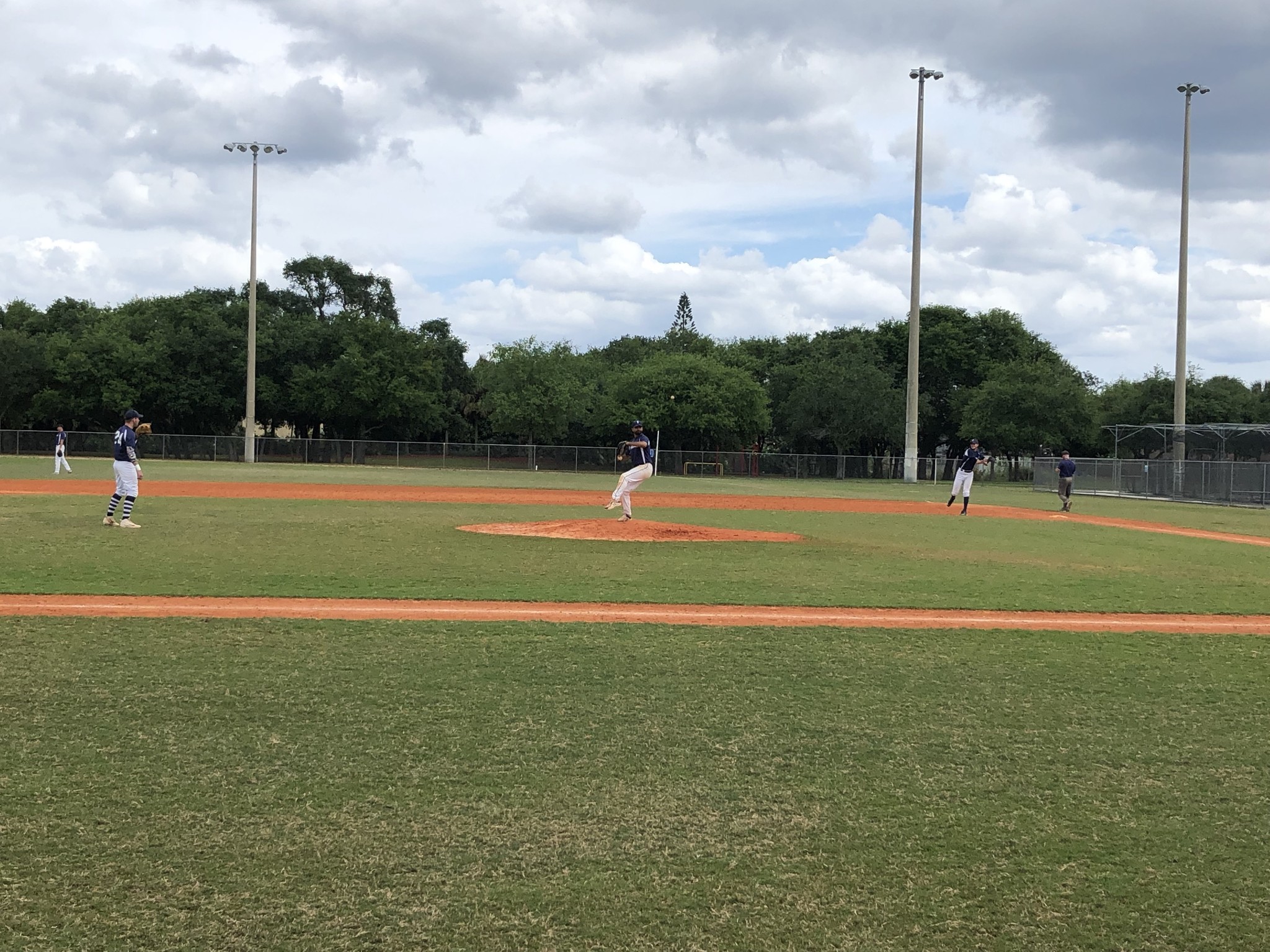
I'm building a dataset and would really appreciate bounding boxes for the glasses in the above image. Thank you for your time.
[970,443,976,445]
[635,426,642,429]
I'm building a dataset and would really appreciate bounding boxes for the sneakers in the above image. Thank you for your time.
[604,501,621,510]
[103,517,120,526]
[120,518,141,528]
[618,514,632,522]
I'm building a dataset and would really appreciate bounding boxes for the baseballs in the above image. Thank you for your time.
[618,455,623,460]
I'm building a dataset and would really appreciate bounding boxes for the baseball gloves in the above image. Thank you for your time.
[57,449,63,457]
[617,440,627,456]
[982,455,995,466]
[135,422,152,436]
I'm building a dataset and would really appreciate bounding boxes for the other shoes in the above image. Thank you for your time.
[52,472,59,475]
[1066,501,1072,511]
[1060,508,1066,512]
[68,470,72,473]
[947,498,955,507]
[960,510,966,516]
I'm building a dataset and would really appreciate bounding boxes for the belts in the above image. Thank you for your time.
[961,469,972,473]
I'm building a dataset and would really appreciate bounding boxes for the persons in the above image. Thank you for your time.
[947,439,988,516]
[605,421,653,522]
[103,410,144,528]
[52,424,72,475]
[1054,450,1076,511]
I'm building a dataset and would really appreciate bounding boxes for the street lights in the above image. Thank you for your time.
[1170,81,1210,487]
[224,141,287,464]
[903,68,944,485]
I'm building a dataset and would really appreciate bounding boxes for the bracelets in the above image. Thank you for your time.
[135,464,141,471]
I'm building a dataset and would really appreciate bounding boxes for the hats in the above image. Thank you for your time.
[970,439,978,444]
[1061,450,1069,455]
[56,424,63,427]
[632,420,642,427]
[124,410,144,419]
